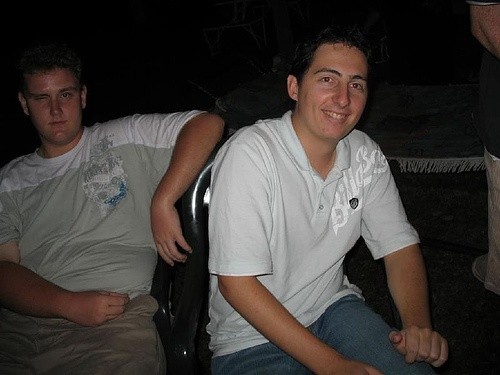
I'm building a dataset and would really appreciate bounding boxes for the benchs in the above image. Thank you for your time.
[204,84,488,252]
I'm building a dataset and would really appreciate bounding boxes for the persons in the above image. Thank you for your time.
[0,44,225,374]
[206,28,447,374]
[463,0,500,295]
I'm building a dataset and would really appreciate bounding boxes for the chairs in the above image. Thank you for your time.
[151,135,402,375]
